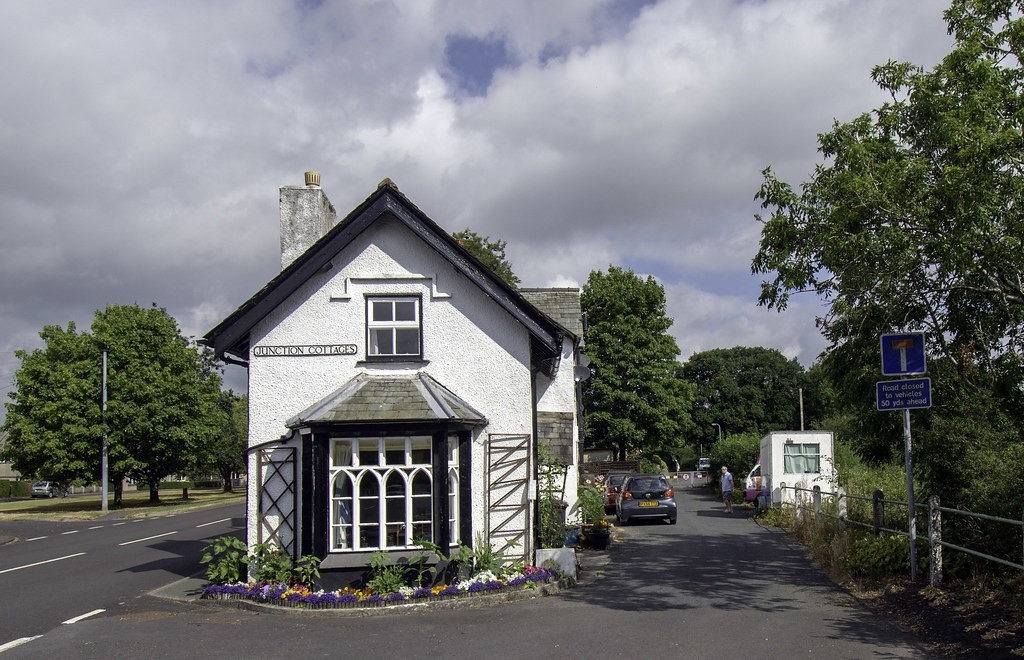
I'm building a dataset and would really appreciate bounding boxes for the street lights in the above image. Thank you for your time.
[712,423,721,444]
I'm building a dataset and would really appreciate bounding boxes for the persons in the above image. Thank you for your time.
[721,466,734,513]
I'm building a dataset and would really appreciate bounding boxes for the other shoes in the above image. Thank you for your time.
[724,509,733,513]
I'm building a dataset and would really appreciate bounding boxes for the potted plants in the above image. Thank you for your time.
[576,484,611,551]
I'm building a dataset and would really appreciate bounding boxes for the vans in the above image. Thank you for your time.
[743,458,839,508]
[695,458,711,473]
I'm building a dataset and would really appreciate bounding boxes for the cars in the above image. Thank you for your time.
[31,480,68,497]
[603,469,638,485]
[603,474,631,514]
[615,474,677,527]
